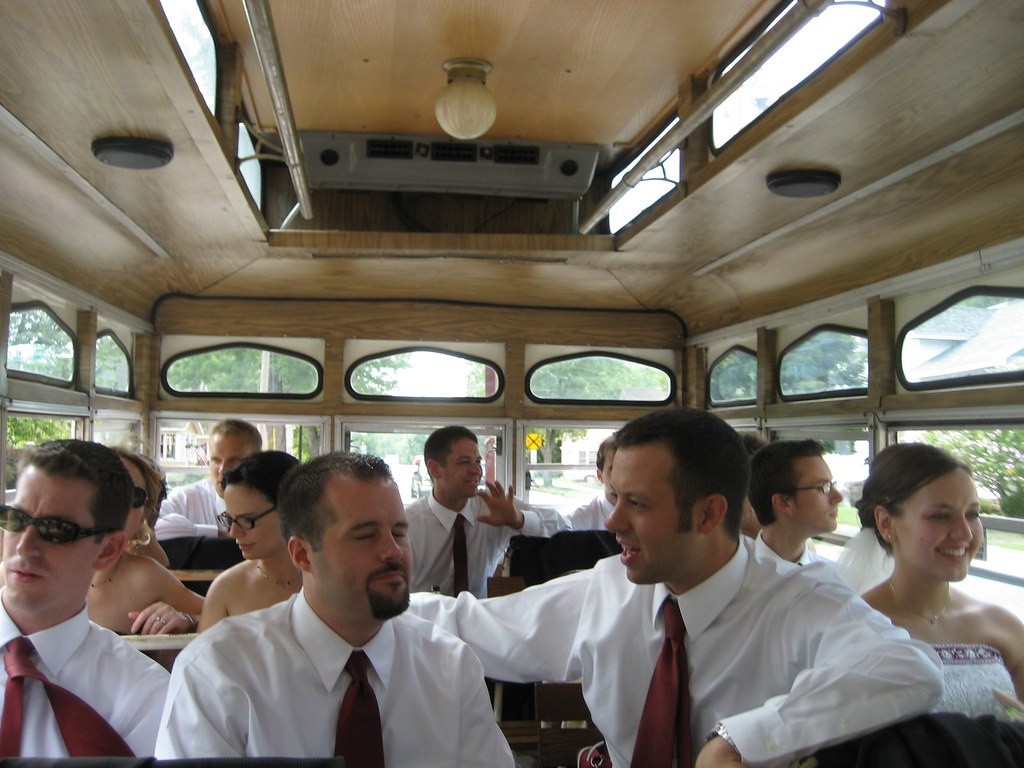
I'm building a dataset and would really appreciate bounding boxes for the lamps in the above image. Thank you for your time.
[434,57,498,141]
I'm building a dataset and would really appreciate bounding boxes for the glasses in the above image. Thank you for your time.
[216,504,277,530]
[130,487,149,508]
[783,481,837,495]
[0,504,118,544]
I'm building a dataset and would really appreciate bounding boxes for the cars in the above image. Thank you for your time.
[410,455,432,500]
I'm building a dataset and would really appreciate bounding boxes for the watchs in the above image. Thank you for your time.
[704,722,741,758]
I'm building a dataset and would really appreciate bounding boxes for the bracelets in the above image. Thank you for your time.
[186,612,194,623]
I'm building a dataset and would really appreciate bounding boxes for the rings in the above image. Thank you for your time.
[156,617,166,624]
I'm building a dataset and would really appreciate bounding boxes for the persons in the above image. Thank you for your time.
[840,442,1024,720]
[196,449,304,633]
[403,426,573,600]
[0,439,171,757]
[750,437,844,565]
[151,451,520,767]
[127,451,170,568]
[154,417,263,540]
[738,430,770,541]
[402,404,944,768]
[85,446,205,673]
[567,434,621,535]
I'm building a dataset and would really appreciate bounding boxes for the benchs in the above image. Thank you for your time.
[500,530,622,768]
[157,537,247,597]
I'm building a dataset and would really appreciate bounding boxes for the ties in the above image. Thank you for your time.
[454,514,468,598]
[630,591,692,768]
[335,650,385,768]
[0,637,136,758]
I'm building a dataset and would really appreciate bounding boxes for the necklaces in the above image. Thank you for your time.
[89,553,125,587]
[742,497,753,531]
[888,580,953,624]
[254,562,303,587]
